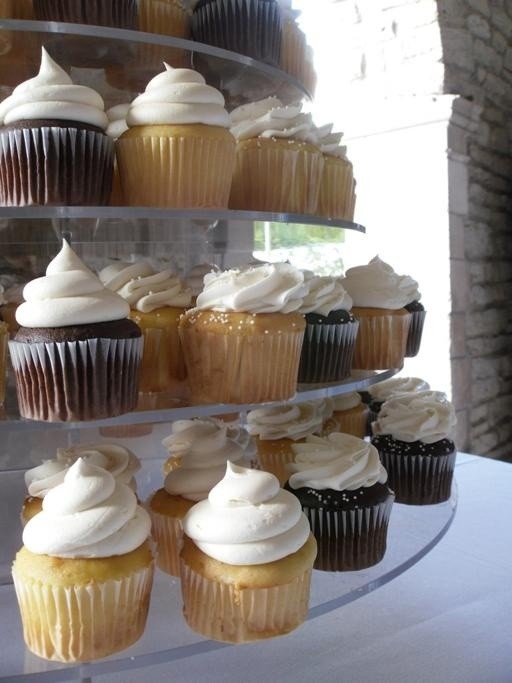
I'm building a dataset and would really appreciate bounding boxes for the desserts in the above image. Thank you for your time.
[0,235,457,664]
[0,0,357,222]
